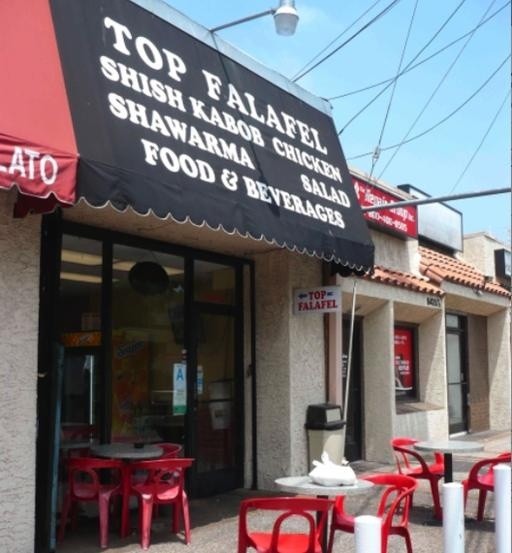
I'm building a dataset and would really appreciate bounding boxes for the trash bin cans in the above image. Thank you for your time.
[305,405,347,474]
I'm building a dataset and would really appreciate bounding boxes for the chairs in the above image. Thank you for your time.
[391,437,445,521]
[462,451,510,526]
[330,470,417,552]
[58,436,195,550]
[238,497,333,552]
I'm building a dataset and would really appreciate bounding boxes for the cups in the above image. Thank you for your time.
[133,441,144,448]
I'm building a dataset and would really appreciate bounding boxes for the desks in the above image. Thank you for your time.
[414,439,485,484]
[276,472,375,552]
